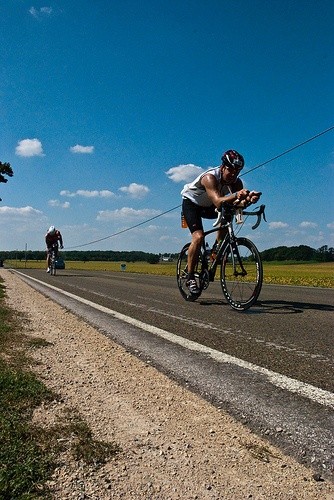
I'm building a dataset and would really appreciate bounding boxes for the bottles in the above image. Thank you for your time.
[205,242,213,263]
[210,251,217,263]
[180,209,188,228]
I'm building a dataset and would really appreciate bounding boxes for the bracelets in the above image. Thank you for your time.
[235,192,237,199]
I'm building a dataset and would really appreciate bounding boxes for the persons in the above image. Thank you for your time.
[45,226,64,273]
[180,150,259,293]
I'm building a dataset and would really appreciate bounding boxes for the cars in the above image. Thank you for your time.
[163,257,173,262]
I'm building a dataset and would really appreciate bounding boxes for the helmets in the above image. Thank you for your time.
[221,150,245,170]
[48,226,56,236]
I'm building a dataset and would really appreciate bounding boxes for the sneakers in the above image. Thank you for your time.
[210,246,219,264]
[185,279,199,294]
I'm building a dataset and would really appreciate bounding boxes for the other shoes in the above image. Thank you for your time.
[47,267,50,273]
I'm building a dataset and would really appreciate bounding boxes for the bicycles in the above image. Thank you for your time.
[48,244,64,276]
[176,202,268,312]
